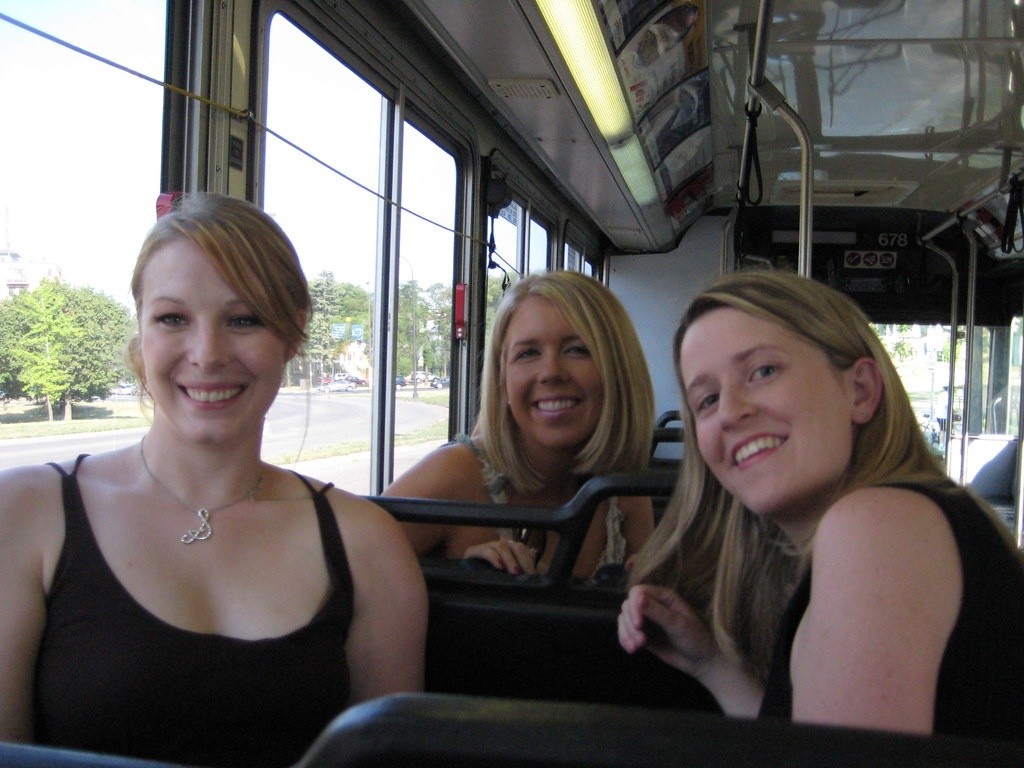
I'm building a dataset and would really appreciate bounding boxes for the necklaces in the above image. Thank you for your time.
[140,442,264,544]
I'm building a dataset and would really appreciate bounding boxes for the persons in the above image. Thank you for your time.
[380,271,654,582]
[1,189,427,768]
[616,270,1024,742]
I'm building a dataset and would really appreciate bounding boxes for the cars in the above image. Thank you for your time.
[318,372,366,392]
[395,371,451,389]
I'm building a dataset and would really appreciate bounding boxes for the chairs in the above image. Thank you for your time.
[0,407,1024,768]
